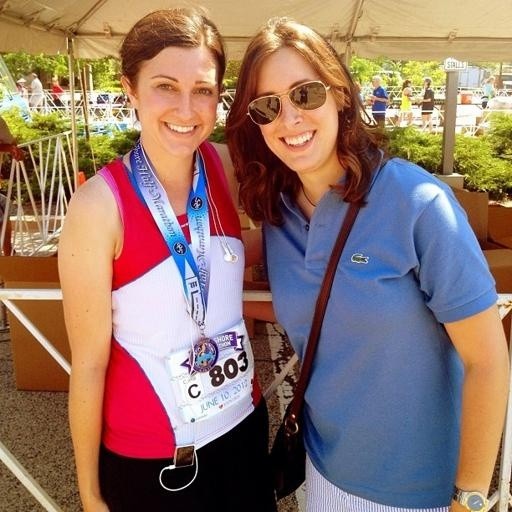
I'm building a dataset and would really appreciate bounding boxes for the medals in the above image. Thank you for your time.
[190,337,220,373]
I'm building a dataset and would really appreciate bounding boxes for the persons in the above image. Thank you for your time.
[368,76,389,126]
[16,79,29,108]
[227,14,512,512]
[415,77,434,132]
[29,72,44,111]
[1,115,28,256]
[56,4,278,512]
[396,81,420,128]
[50,76,64,112]
[484,77,497,99]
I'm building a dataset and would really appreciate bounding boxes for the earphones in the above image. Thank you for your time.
[227,243,238,262]
[221,243,233,262]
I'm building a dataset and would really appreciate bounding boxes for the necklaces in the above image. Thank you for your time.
[303,183,317,208]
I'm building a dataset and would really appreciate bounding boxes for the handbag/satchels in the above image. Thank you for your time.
[270,410,306,502]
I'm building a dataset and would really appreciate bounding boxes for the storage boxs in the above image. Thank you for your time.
[449,188,512,351]
[0,251,72,392]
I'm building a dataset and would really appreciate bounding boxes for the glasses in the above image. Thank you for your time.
[246,81,331,127]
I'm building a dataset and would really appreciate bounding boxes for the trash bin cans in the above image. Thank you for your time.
[461,94,473,104]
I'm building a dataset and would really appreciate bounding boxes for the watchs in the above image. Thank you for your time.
[452,485,489,512]
